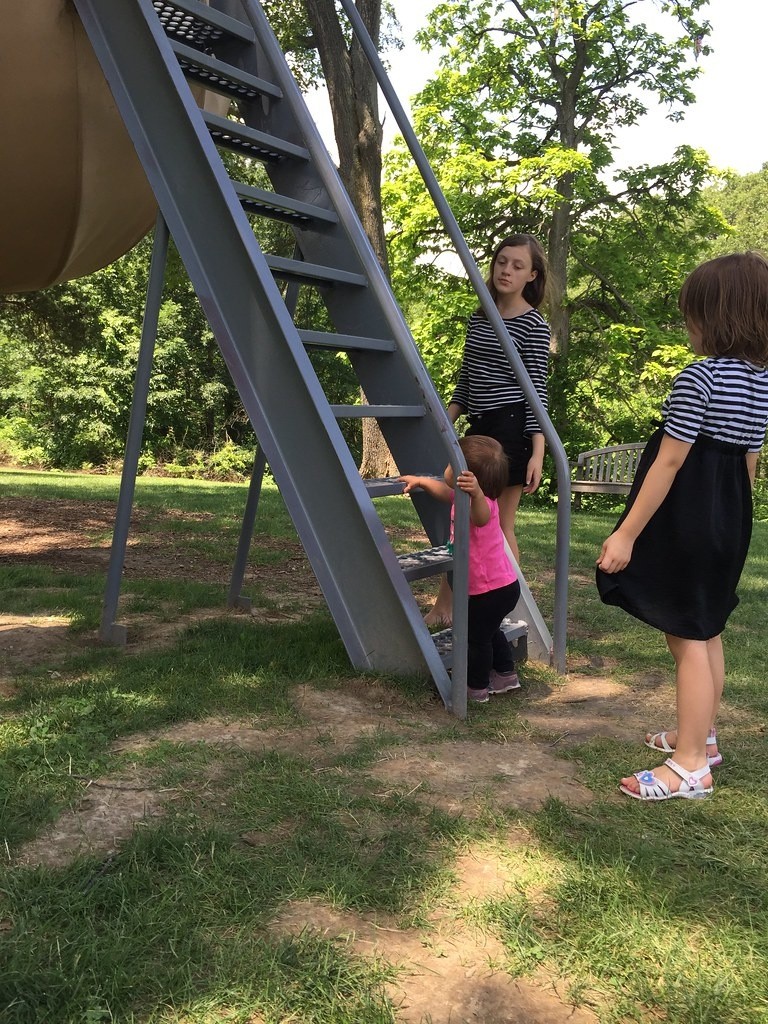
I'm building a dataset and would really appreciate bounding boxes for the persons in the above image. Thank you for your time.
[392,434,524,706]
[416,233,552,631]
[591,249,767,807]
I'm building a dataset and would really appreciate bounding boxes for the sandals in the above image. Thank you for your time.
[620,758,714,800]
[644,727,722,767]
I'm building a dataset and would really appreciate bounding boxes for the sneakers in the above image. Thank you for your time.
[487,669,521,694]
[466,686,489,703]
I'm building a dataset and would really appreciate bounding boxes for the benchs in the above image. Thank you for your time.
[564,442,648,509]
